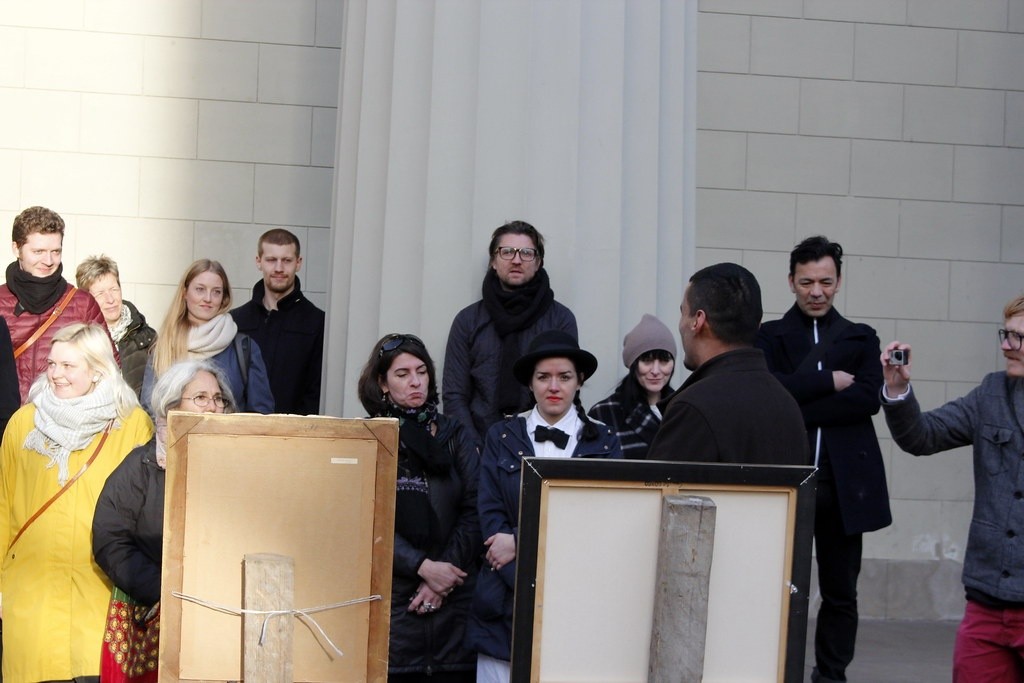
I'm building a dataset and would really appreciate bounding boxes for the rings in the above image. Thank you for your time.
[423,603,431,612]
[431,606,437,609]
[449,588,453,592]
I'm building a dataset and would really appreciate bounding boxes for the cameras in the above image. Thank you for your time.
[888,350,909,366]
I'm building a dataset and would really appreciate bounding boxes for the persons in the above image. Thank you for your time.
[442,221,579,455]
[76,255,158,399]
[756,236,892,683]
[0,324,154,683]
[879,294,1024,683]
[141,258,276,425]
[588,313,677,460]
[228,229,326,416]
[0,206,122,408]
[91,359,236,683]
[357,333,481,683]
[646,262,811,466]
[465,330,624,683]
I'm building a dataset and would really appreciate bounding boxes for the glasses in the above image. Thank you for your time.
[379,334,425,357]
[999,329,1024,350]
[494,246,539,261]
[181,396,230,408]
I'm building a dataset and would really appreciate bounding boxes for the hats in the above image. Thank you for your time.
[623,314,677,369]
[515,331,597,381]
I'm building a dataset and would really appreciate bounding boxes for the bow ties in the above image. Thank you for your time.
[535,425,570,450]
